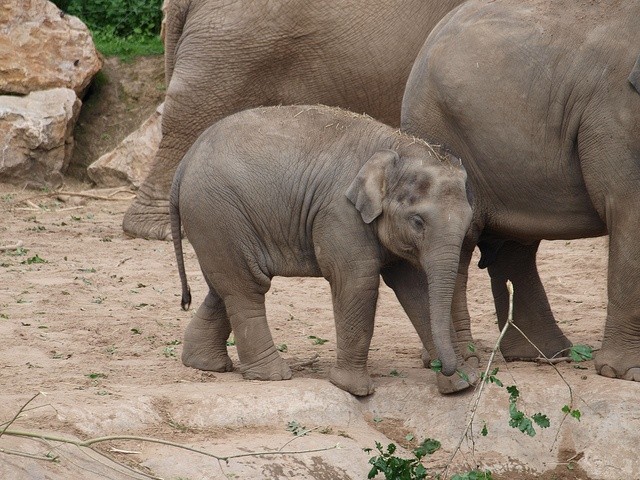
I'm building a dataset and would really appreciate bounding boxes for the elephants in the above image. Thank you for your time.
[169,104,482,396]
[121,0,469,242]
[392,0,640,383]
